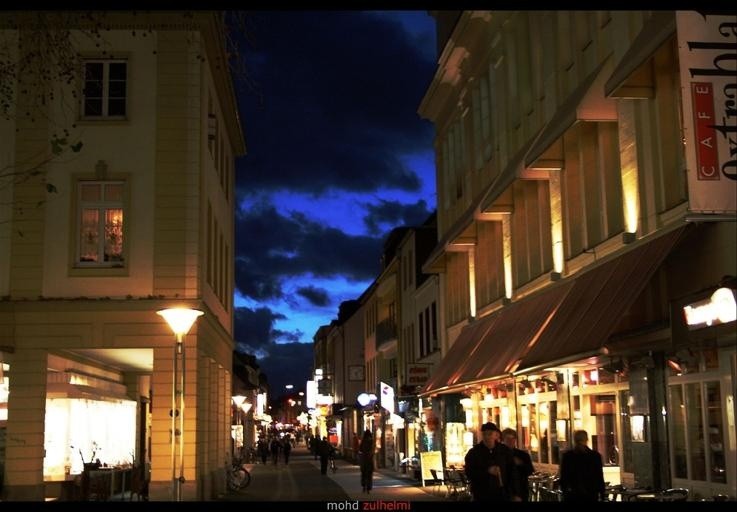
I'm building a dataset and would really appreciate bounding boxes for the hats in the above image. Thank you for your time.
[482,422,500,431]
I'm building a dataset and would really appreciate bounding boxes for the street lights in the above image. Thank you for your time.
[231,393,248,469]
[153,303,205,500]
[240,404,253,456]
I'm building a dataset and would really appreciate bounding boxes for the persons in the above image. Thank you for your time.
[309,435,333,475]
[261,438,292,464]
[466,422,534,501]
[560,429,606,501]
[360,429,375,494]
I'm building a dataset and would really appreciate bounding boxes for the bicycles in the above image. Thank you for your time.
[329,455,337,474]
[228,454,250,493]
